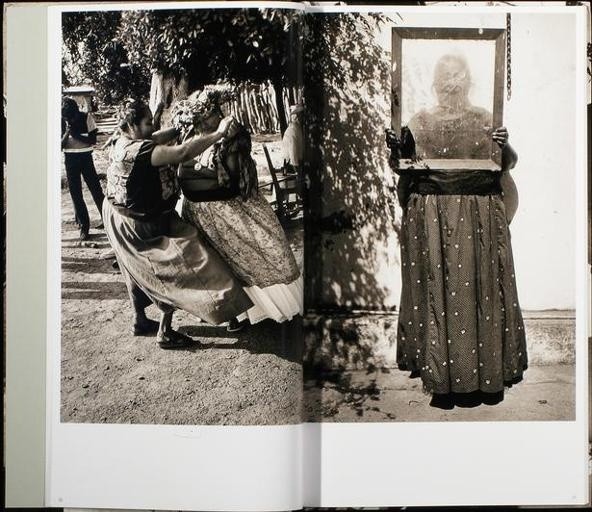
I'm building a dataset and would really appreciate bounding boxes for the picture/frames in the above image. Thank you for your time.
[391,28,506,171]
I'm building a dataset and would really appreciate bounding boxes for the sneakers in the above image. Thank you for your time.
[80,221,104,241]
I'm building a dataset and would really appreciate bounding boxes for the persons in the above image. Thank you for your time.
[385,54,530,396]
[169,94,303,332]
[62,99,105,240]
[102,97,246,349]
[282,104,303,198]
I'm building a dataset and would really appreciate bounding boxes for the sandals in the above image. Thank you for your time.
[226,320,247,332]
[157,330,200,349]
[133,319,160,336]
